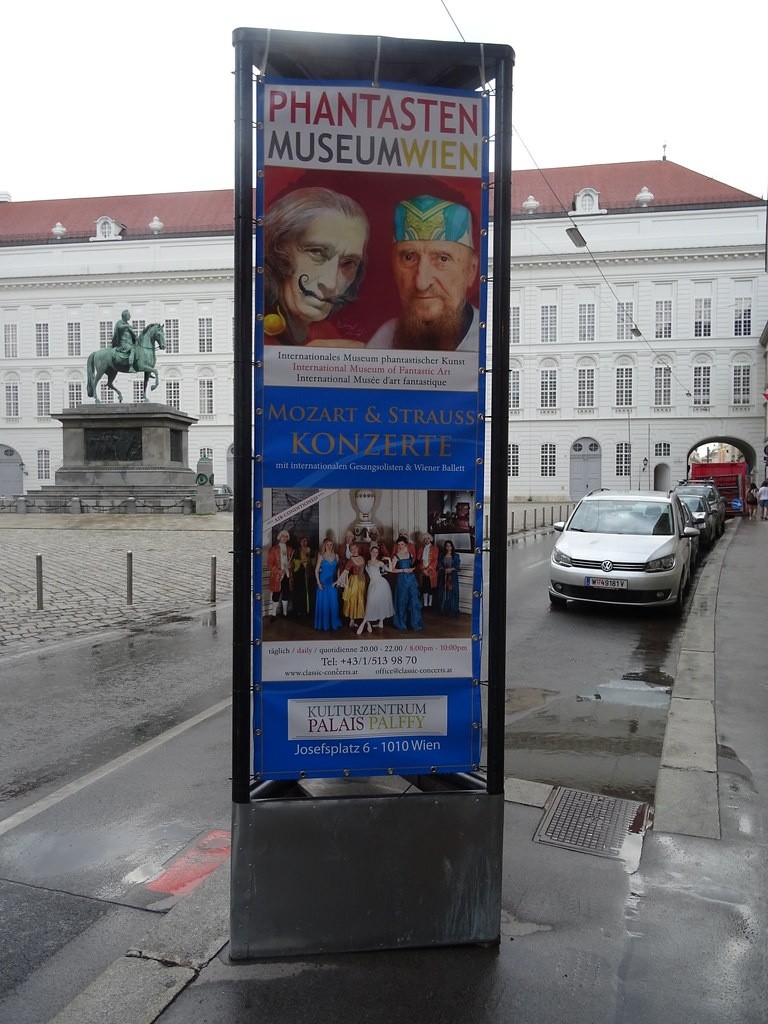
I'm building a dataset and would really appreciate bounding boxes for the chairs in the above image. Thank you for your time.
[628,504,669,535]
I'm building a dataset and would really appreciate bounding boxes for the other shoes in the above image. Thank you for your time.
[760,516,767,520]
[270,616,276,622]
[367,623,373,633]
[282,614,295,619]
[357,623,364,635]
[423,606,432,613]
[372,624,383,628]
[349,620,358,628]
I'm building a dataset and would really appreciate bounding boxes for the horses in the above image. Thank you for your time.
[86,323,168,404]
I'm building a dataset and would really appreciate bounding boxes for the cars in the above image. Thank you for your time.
[670,479,727,553]
[541,487,701,615]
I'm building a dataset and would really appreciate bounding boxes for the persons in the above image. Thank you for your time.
[111,310,137,373]
[744,483,759,520]
[755,480,768,520]
[269,531,460,634]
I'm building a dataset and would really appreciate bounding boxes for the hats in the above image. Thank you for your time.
[392,195,475,251]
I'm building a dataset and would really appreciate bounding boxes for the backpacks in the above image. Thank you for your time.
[746,491,754,503]
